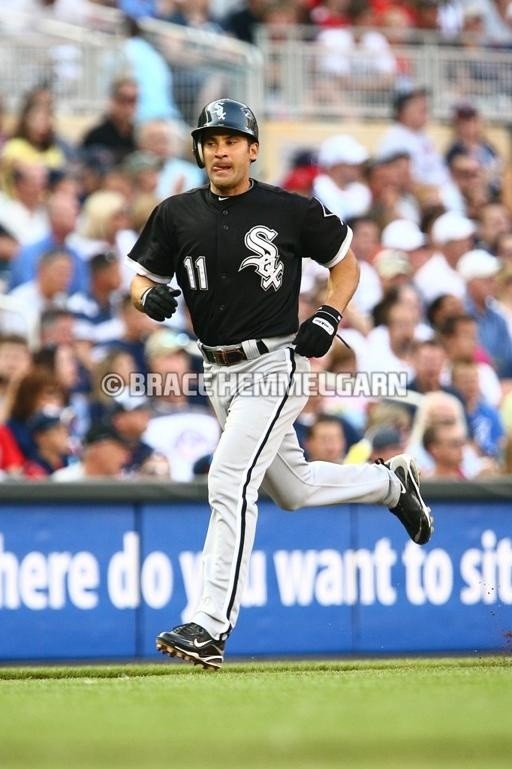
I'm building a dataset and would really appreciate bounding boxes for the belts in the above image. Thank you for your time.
[201,342,270,365]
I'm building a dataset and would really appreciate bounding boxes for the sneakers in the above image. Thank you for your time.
[155,622,231,672]
[382,455,431,545]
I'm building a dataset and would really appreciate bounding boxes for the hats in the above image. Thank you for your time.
[145,332,192,363]
[316,134,500,283]
[28,387,153,444]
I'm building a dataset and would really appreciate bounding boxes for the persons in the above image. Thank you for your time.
[0,1,512,482]
[125,100,435,671]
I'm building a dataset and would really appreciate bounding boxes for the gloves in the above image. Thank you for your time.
[141,285,182,320]
[293,305,343,357]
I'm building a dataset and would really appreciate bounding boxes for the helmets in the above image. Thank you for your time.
[191,100,259,169]
